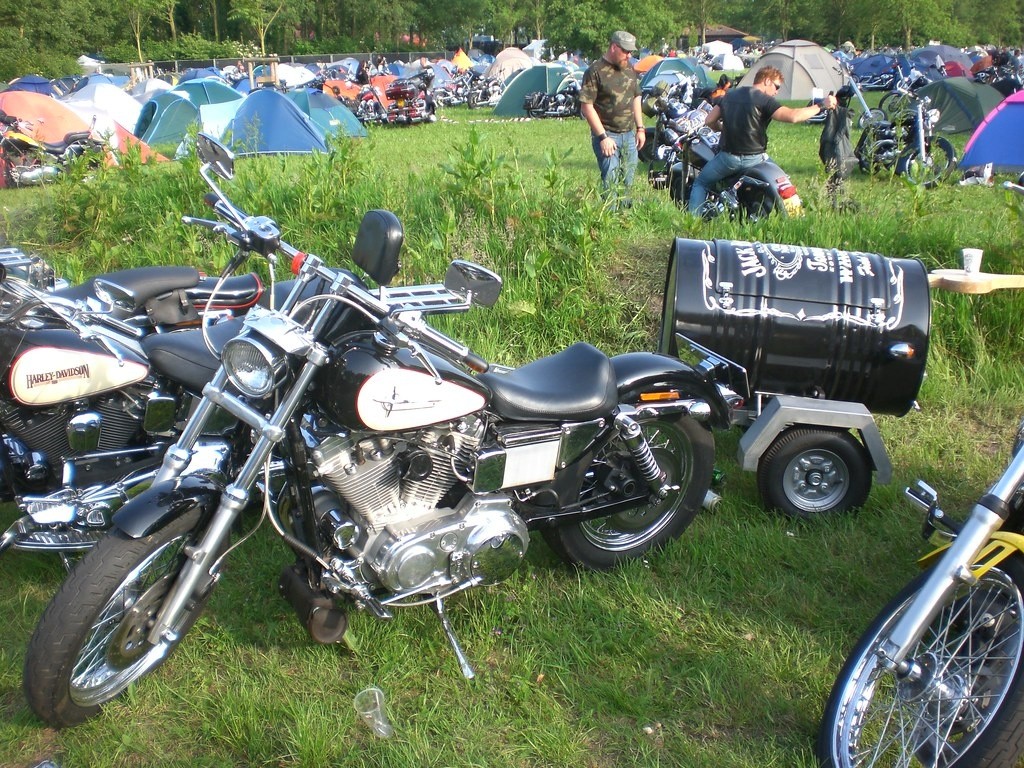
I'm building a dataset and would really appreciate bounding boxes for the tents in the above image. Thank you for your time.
[733,40,1024,174]
[0,55,359,187]
[628,39,744,94]
[383,46,589,117]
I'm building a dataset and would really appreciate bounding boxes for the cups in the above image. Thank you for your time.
[962,246,983,279]
[354,687,394,741]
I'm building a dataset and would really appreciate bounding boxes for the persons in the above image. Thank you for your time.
[688,63,837,221]
[992,47,1000,64]
[658,49,667,58]
[579,30,646,214]
[998,46,1013,67]
[356,60,388,85]
[668,48,677,57]
[1011,49,1021,68]
[558,51,567,61]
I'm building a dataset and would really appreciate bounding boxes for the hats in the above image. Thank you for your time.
[612,31,638,51]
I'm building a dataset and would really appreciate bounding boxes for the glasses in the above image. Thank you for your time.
[770,79,781,90]
[617,44,632,54]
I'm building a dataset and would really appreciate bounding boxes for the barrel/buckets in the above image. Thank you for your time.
[660,239,933,419]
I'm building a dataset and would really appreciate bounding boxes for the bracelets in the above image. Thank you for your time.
[598,132,610,142]
[637,126,645,132]
[815,101,827,112]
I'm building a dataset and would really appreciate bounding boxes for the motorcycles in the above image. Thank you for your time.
[0,110,106,189]
[0,246,471,575]
[853,73,958,192]
[668,99,807,227]
[249,40,1024,129]
[24,129,750,730]
[816,415,1023,768]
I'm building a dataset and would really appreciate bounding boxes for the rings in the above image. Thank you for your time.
[833,102,835,105]
